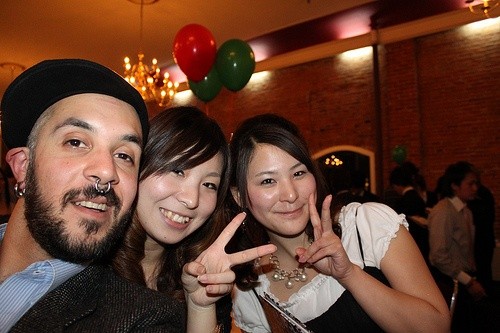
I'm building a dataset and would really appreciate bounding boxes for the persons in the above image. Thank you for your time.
[0,59,187,333]
[107,106,497,333]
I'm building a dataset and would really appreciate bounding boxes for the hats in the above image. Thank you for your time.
[0,58,149,152]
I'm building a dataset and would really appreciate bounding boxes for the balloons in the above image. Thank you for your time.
[173,24,255,104]
[390,144,406,165]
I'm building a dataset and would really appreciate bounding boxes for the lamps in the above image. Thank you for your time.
[123,0,179,108]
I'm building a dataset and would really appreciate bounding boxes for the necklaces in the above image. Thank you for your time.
[268,240,309,288]
